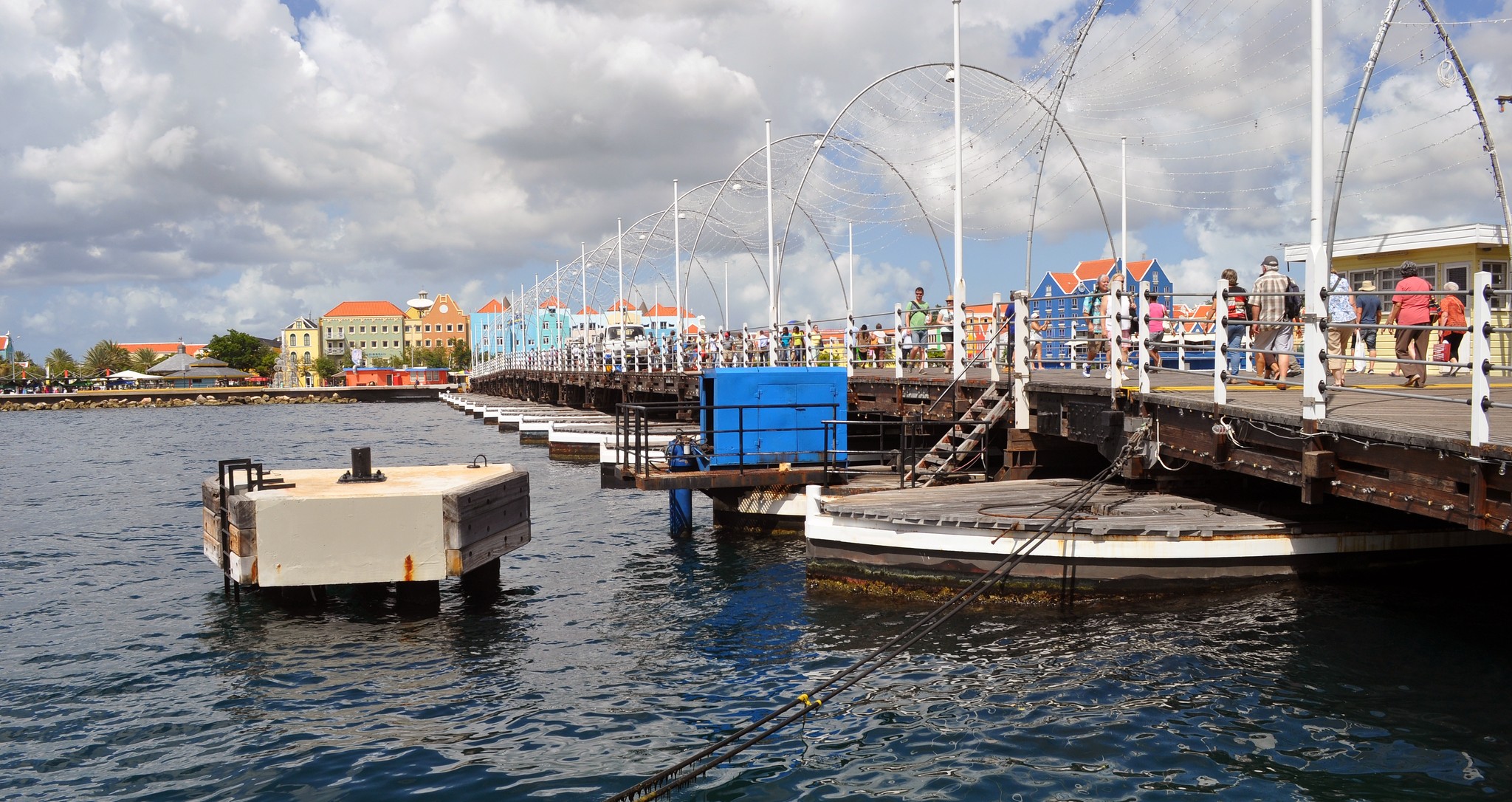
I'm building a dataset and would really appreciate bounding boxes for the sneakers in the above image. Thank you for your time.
[1449,365,1462,376]
[1346,369,1359,374]
[1404,374,1420,385]
[1442,372,1456,377]
[1227,378,1237,384]
[903,363,954,375]
[1002,366,1012,373]
[1105,366,1112,380]
[1121,371,1129,380]
[852,363,884,369]
[1327,370,1332,376]
[1082,363,1091,378]
[1367,369,1375,374]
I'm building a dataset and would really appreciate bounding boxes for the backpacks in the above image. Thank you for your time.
[1282,276,1302,319]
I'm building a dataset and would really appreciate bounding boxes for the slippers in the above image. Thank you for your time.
[1149,370,1158,374]
[1388,371,1400,377]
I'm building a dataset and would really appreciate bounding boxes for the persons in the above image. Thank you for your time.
[1000,290,1015,373]
[905,287,932,376]
[1253,273,1303,385]
[1346,280,1382,375]
[215,366,474,394]
[1388,260,1433,388]
[1380,301,1416,377]
[0,360,176,395]
[1327,267,1357,392]
[1247,255,1296,391]
[1029,312,1051,371]
[1202,269,1252,385]
[1438,281,1467,377]
[972,362,988,369]
[1100,273,1137,380]
[1135,291,1176,374]
[1081,274,1112,378]
[518,320,915,371]
[936,295,969,374]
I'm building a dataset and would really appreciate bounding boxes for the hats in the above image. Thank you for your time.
[1260,256,1278,266]
[1031,313,1040,319]
[1358,281,1376,291]
[724,333,729,336]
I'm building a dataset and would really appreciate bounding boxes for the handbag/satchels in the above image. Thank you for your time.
[1242,288,1253,326]
[1160,304,1173,333]
[1029,330,1036,352]
[1433,333,1451,362]
[1425,280,1441,323]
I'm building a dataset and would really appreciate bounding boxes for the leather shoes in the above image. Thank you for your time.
[1286,371,1301,377]
[1248,380,1265,386]
[1276,383,1286,390]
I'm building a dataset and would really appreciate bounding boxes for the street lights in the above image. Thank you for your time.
[408,323,421,369]
[5,334,21,382]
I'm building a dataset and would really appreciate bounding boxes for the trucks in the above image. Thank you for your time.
[587,324,649,369]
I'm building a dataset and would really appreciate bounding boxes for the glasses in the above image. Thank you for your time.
[1010,291,1013,297]
[945,300,952,302]
[1113,280,1124,284]
[916,293,922,295]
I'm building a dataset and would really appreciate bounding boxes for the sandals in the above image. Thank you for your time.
[1272,369,1280,384]
[1340,378,1345,386]
[1156,358,1162,372]
[1325,382,1342,392]
